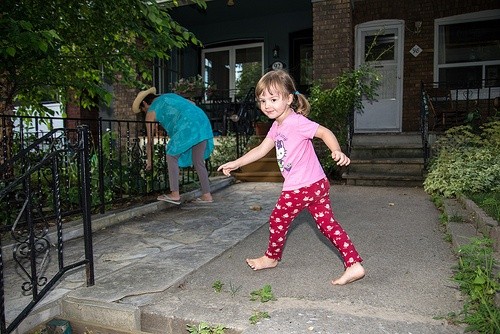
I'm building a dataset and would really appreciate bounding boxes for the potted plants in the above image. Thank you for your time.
[206,80,228,97]
[255,116,271,135]
[171,74,205,98]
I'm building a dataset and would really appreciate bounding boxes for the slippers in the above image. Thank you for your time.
[191,197,214,204]
[157,193,181,205]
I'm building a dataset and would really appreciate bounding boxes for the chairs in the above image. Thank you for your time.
[425,86,468,130]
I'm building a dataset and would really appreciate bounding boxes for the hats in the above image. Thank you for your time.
[132,87,156,114]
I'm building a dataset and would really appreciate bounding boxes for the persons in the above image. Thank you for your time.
[217,69,365,284]
[132,88,215,205]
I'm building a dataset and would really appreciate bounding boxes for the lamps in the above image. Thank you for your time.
[272,45,280,58]
[413,21,422,34]
